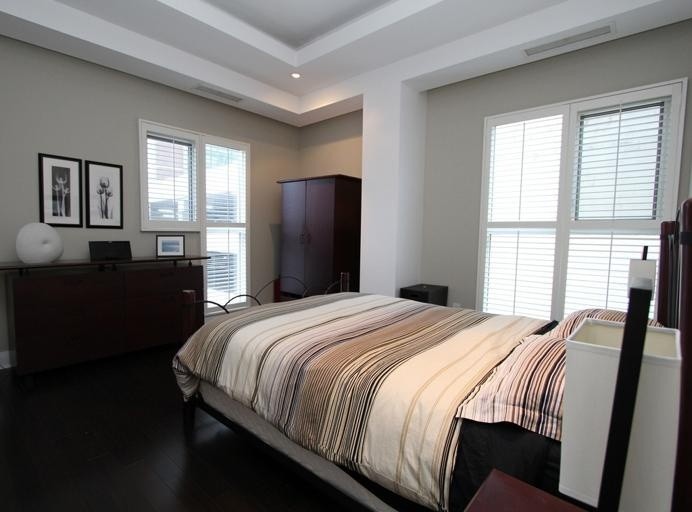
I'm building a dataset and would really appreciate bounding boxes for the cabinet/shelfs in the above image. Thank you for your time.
[399,282,448,306]
[0,252,205,376]
[276,175,359,300]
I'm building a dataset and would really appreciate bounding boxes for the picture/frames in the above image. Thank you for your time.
[82,159,124,231]
[154,234,186,257]
[39,151,84,229]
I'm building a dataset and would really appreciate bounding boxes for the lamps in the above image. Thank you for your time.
[559,246,681,511]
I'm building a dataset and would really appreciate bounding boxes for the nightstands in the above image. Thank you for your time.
[460,466,588,510]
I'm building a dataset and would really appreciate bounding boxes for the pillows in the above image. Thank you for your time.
[456,335,565,442]
[545,306,668,341]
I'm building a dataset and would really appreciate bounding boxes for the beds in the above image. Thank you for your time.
[174,270,687,511]
